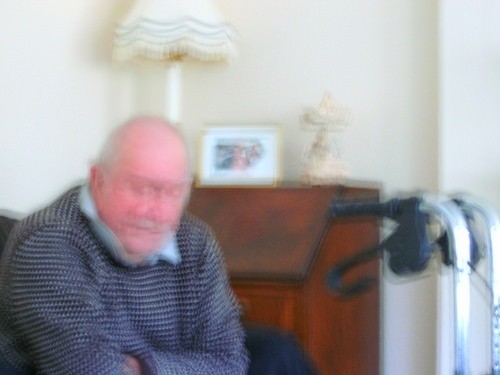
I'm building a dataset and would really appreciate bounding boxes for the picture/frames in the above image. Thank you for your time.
[193,122,288,191]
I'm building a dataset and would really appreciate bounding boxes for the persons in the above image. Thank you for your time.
[0,115,249,374]
[216,137,266,170]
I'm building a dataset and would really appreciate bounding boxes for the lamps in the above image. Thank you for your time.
[111,0,238,128]
